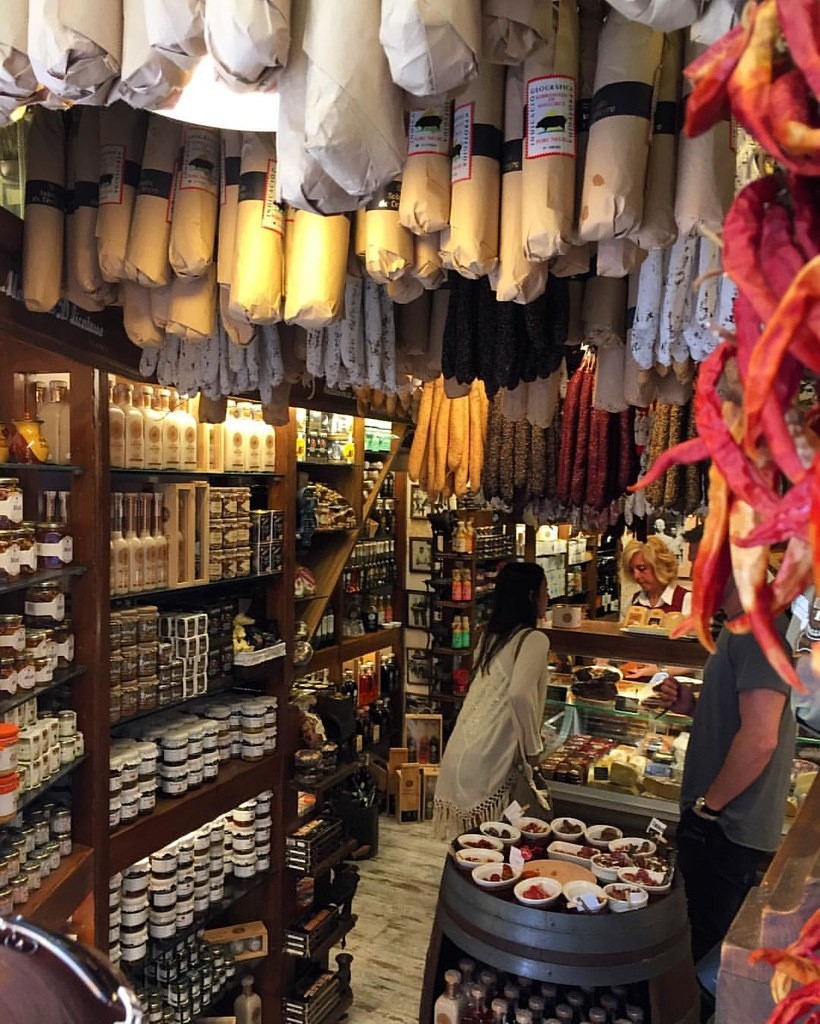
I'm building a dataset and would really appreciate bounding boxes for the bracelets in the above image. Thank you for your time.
[659,665,668,673]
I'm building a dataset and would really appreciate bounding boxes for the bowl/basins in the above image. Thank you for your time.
[454,816,675,913]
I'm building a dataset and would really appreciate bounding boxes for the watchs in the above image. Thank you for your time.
[695,797,720,817]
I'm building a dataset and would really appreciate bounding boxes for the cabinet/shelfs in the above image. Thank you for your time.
[0,320,820,1024]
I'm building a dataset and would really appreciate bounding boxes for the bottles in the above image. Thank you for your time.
[433,957,644,1024]
[42,491,169,595]
[453,615,471,649]
[597,574,619,617]
[313,460,398,652]
[452,569,472,602]
[234,976,261,1024]
[453,521,476,553]
[290,653,438,822]
[34,378,275,472]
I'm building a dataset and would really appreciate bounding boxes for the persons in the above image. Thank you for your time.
[675,527,797,1024]
[592,536,708,678]
[434,561,551,844]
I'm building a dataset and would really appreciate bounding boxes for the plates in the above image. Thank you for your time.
[515,859,597,884]
